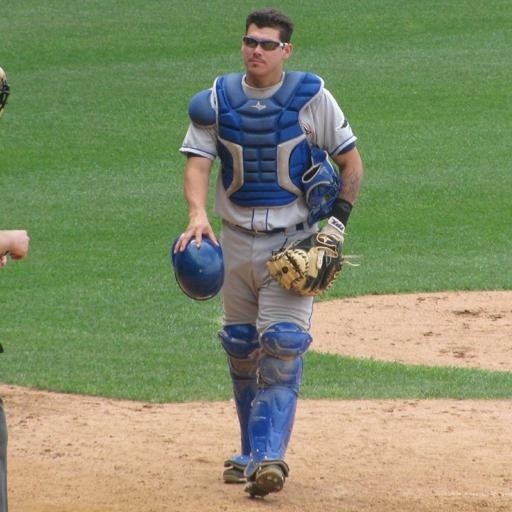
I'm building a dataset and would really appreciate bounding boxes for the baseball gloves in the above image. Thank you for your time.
[265,215,346,296]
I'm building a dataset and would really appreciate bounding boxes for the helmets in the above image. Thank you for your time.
[171,230,226,301]
[0,65,10,116]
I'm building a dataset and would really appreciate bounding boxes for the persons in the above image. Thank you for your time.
[171,9,364,498]
[1,227,32,511]
[1,65,10,116]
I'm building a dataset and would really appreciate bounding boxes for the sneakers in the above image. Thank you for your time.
[224,467,246,484]
[243,463,288,498]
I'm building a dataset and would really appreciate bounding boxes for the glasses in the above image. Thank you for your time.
[242,36,288,51]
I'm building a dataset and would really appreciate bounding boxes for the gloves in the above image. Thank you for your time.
[287,215,345,294]
